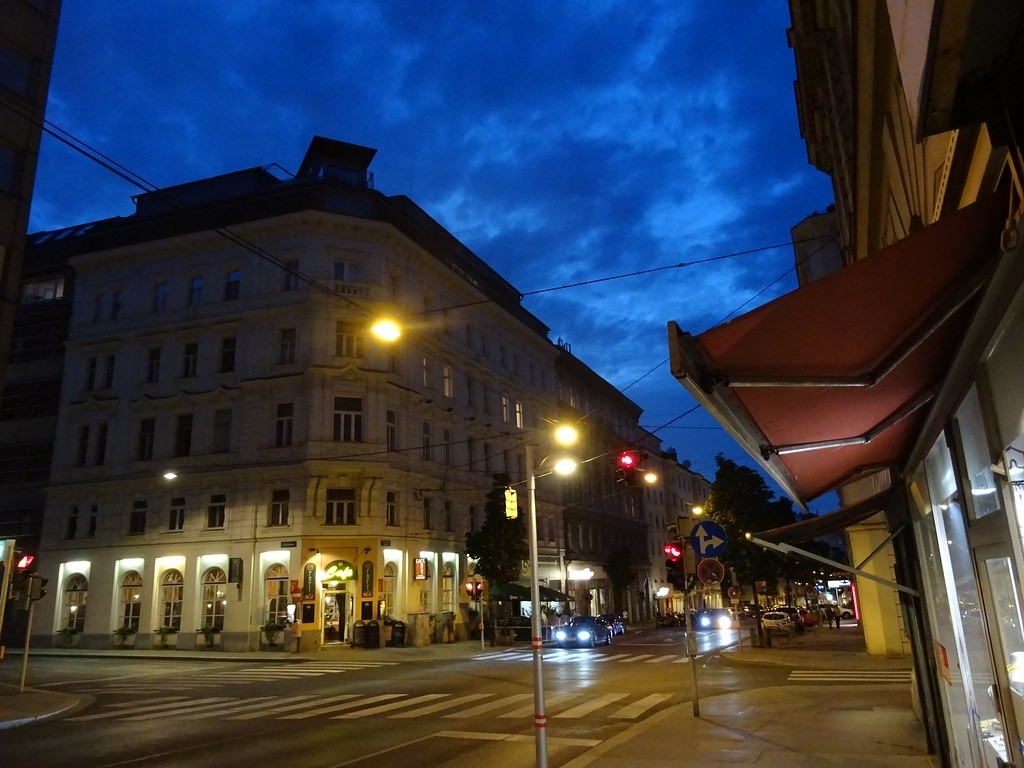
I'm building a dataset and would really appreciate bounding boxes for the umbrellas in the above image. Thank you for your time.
[489,582,575,601]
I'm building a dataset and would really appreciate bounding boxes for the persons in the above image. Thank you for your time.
[825,605,841,630]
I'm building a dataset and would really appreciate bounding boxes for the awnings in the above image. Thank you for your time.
[669,188,1012,514]
[744,483,897,552]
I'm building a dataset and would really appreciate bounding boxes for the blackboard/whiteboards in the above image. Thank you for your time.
[366,626,379,647]
[353,627,365,646]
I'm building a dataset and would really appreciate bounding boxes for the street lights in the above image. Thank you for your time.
[525,443,578,767]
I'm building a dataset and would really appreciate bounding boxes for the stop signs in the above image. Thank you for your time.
[291,587,302,603]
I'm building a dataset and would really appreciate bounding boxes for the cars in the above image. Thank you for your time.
[594,613,625,636]
[655,612,681,628]
[726,602,855,635]
[549,614,614,648]
[693,608,734,629]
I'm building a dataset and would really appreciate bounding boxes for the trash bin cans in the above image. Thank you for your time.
[391,621,406,647]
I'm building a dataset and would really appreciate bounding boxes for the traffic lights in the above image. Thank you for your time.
[465,582,473,596]
[475,581,482,596]
[620,451,637,483]
[15,555,35,570]
[30,577,49,600]
[663,542,684,562]
[499,489,518,519]
